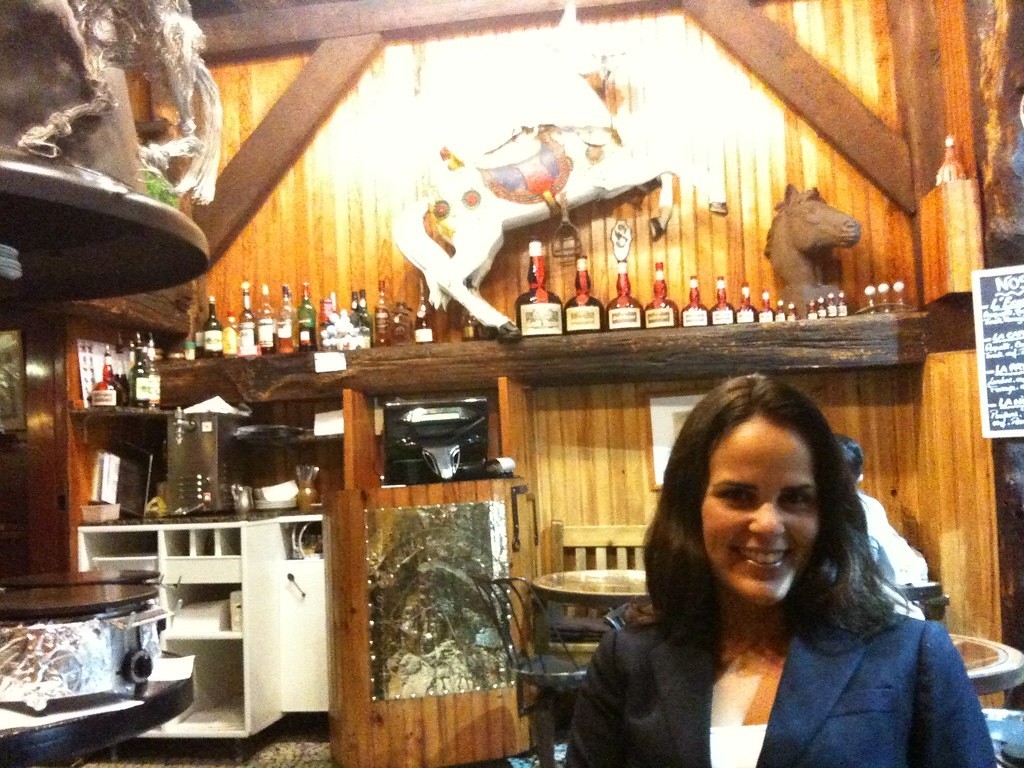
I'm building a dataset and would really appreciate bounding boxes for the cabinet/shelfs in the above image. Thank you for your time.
[72,512,333,763]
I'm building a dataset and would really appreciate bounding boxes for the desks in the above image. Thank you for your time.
[533,564,651,609]
[946,633,1024,698]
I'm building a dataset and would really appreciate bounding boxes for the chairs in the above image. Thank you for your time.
[544,517,650,691]
[468,566,596,768]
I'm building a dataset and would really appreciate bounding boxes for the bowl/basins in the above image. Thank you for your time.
[80,502,121,522]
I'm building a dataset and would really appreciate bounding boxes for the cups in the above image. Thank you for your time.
[232,484,254,513]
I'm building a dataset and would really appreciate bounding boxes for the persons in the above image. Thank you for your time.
[563,372,1000,767]
[827,433,931,622]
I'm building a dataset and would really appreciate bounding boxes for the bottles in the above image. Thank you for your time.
[736,283,757,324]
[936,135,966,184]
[826,292,837,317]
[806,300,817,319]
[757,289,775,322]
[604,261,644,331]
[296,465,319,513]
[91,332,160,409]
[709,276,736,326]
[837,290,847,317]
[182,277,486,360]
[787,303,796,321]
[644,263,679,329]
[515,240,564,337]
[817,296,826,318]
[564,256,604,335]
[680,276,709,327]
[775,300,786,322]
